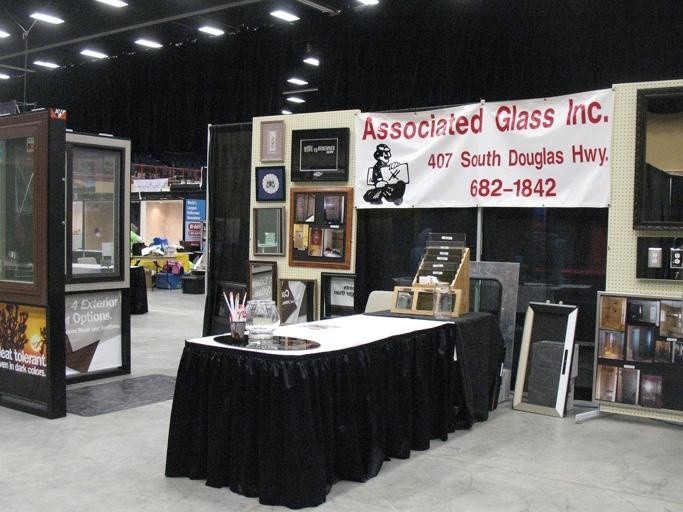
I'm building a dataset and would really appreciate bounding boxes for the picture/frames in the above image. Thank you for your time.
[249,120,359,327]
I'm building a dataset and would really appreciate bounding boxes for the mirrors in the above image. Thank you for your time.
[634,87,683,231]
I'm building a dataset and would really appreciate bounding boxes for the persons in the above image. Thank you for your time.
[130,222,143,260]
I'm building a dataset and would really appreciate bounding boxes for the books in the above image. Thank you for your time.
[615,367,640,406]
[597,294,625,332]
[628,298,659,327]
[658,296,682,340]
[255,167,285,201]
[254,207,281,254]
[638,374,664,410]
[598,329,624,362]
[592,364,616,403]
[260,121,284,163]
[306,227,324,258]
[653,339,670,364]
[294,192,316,222]
[322,227,345,258]
[323,194,343,223]
[624,324,656,365]
[670,342,682,364]
[292,223,309,250]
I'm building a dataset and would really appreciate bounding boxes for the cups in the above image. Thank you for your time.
[230,321,246,345]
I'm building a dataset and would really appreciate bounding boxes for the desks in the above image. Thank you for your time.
[182,310,501,489]
[131,254,178,292]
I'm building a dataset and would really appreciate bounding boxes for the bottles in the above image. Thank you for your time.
[244,299,281,341]
